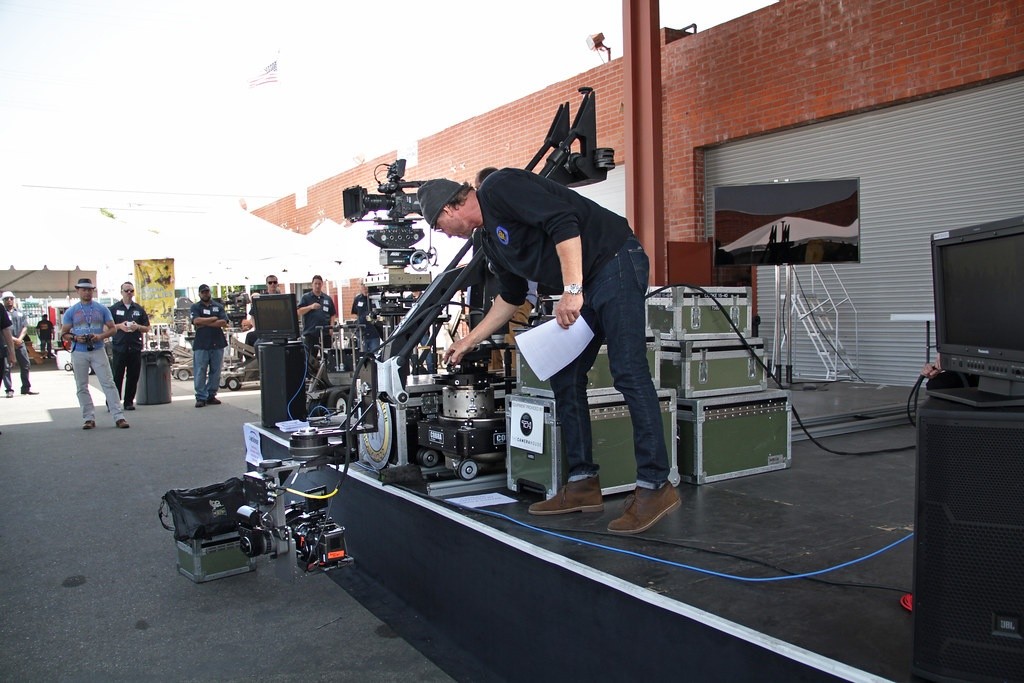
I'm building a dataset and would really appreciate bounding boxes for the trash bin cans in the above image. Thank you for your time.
[135,350,171,404]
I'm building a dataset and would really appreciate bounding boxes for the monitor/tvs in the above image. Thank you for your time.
[713,175,862,266]
[251,293,301,344]
[922,214,1024,408]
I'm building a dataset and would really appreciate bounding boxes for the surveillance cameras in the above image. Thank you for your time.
[584,31,605,48]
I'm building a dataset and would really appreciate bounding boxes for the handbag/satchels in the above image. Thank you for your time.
[157,478,247,541]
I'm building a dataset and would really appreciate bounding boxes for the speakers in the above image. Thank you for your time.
[259,346,305,429]
[910,395,1024,683]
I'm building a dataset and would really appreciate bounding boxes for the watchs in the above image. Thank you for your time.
[563,283,583,294]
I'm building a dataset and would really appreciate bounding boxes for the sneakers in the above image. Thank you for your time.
[607,478,682,535]
[528,475,604,515]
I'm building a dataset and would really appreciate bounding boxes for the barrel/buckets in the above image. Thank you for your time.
[56,350,71,369]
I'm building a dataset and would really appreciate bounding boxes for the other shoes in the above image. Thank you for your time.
[6,392,13,397]
[22,390,39,394]
[205,397,221,405]
[116,419,129,428]
[195,401,205,407]
[124,405,136,410]
[83,420,95,429]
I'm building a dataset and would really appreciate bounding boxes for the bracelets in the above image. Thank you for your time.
[137,325,140,331]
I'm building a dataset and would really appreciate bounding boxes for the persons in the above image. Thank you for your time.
[416,166,680,535]
[61,279,129,429]
[242,275,281,366]
[187,284,229,407]
[297,276,336,378]
[36,314,55,359]
[106,282,150,412]
[351,278,437,374]
[0,291,39,398]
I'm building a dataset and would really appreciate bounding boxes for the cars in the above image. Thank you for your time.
[172,356,195,381]
[220,356,259,391]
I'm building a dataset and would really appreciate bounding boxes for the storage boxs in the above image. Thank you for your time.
[504,283,792,501]
[176,531,256,583]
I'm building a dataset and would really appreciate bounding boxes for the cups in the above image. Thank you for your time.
[127,322,133,332]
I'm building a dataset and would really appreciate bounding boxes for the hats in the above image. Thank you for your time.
[199,284,210,292]
[1,291,15,299]
[74,278,95,288]
[417,179,465,229]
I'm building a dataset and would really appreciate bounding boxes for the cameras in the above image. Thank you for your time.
[82,332,95,352]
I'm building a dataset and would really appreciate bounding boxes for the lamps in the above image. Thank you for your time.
[587,33,611,62]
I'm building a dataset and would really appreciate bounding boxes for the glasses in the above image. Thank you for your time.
[267,281,278,285]
[434,218,443,233]
[121,289,134,293]
